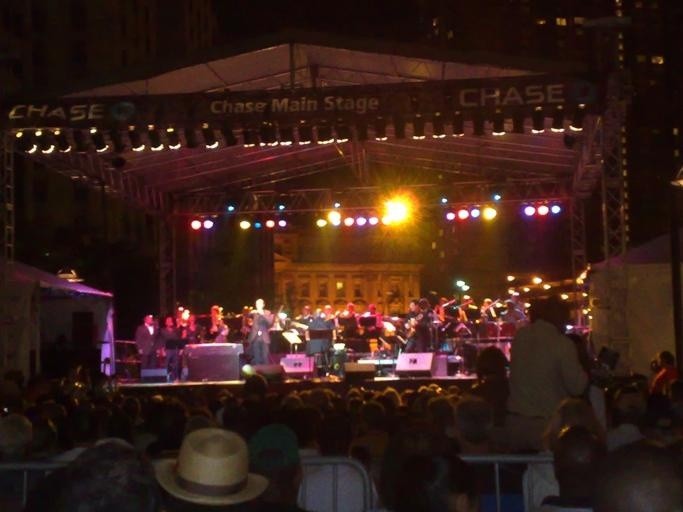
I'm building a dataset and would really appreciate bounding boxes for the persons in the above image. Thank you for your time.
[1,290,682,512]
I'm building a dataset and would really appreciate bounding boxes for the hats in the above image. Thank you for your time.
[152,427,268,505]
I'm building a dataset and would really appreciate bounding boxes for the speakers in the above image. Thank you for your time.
[252,364,290,381]
[141,368,173,383]
[336,362,377,382]
[280,357,320,379]
[448,355,460,376]
[395,352,436,378]
